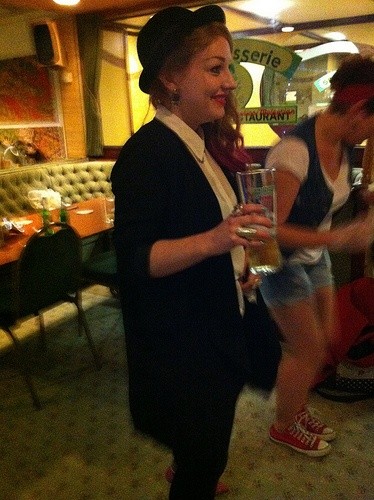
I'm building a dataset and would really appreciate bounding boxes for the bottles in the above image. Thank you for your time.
[41,197,52,236]
[60,196,70,229]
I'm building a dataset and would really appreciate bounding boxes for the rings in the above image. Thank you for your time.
[236,227,258,238]
[230,203,244,216]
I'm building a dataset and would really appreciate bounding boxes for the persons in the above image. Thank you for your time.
[253,51,374,458]
[107,5,286,500]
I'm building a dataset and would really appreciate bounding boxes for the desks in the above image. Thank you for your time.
[0,197,115,267]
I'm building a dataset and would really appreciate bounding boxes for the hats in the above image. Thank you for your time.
[136,4,226,95]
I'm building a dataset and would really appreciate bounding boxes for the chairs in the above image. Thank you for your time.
[76,252,118,335]
[0,222,103,409]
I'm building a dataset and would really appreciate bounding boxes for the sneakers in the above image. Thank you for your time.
[295,404,336,442]
[269,420,331,458]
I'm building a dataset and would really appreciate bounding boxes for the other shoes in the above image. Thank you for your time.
[165,466,229,495]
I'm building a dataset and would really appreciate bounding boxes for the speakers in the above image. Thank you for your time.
[30,19,65,69]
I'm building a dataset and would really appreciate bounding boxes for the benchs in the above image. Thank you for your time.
[0,160,117,218]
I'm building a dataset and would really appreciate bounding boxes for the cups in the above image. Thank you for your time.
[236,167,282,273]
[106,198,114,224]
